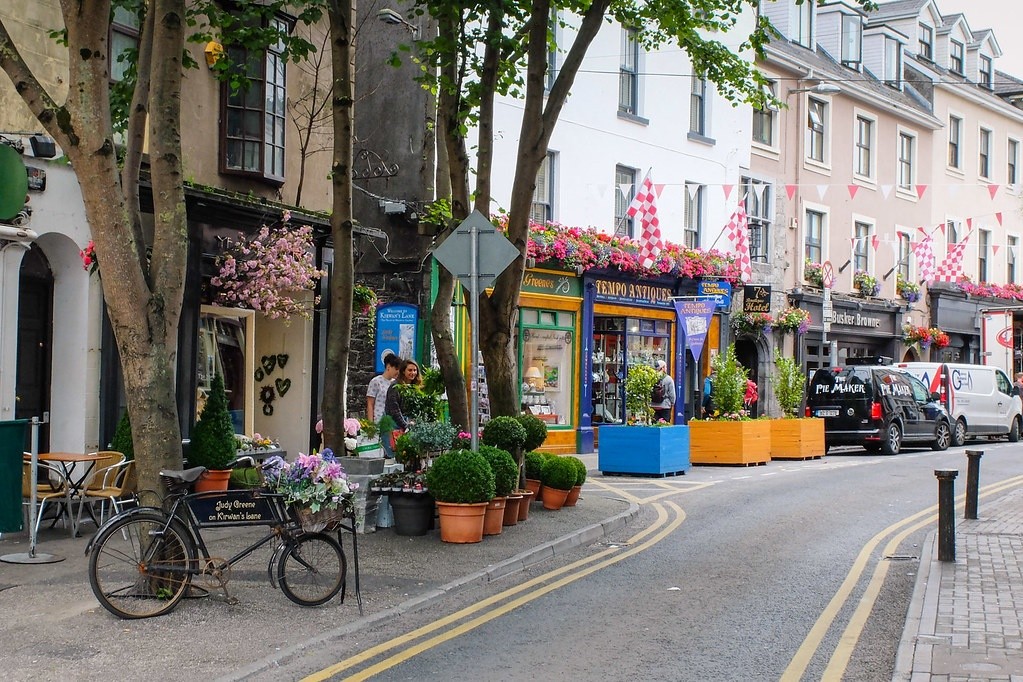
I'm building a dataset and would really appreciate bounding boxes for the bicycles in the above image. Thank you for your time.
[83,456,364,620]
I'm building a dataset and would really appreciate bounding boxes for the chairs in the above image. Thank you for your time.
[22,450,136,540]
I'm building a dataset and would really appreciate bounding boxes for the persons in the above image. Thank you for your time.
[1014,371,1023,440]
[379,357,422,459]
[701,373,715,419]
[648,360,676,425]
[367,353,402,458]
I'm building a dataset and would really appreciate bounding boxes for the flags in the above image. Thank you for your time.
[913,235,935,283]
[723,197,752,283]
[627,171,663,270]
[935,237,967,282]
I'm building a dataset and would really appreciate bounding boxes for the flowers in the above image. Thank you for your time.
[777,305,812,335]
[212,210,330,328]
[316,419,361,450]
[490,213,746,289]
[901,323,950,351]
[79,240,101,280]
[730,311,775,335]
[260,448,359,514]
[451,431,484,450]
[854,269,880,299]
[897,272,922,303]
[237,434,280,450]
[353,284,385,350]
[958,271,1023,302]
[805,258,837,289]
[625,365,670,427]
[690,343,770,421]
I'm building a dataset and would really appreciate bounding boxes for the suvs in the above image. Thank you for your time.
[804,355,952,456]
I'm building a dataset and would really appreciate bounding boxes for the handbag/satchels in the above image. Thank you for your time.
[391,428,405,450]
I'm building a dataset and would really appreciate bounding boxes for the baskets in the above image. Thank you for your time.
[288,494,343,534]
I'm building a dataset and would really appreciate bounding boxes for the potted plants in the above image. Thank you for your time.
[425,415,547,544]
[231,465,260,495]
[188,370,240,499]
[770,344,825,461]
[525,452,587,510]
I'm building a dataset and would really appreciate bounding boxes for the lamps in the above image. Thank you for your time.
[785,84,841,98]
[377,8,421,42]
[524,367,542,392]
[0,131,57,158]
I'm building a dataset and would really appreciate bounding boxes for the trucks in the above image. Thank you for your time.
[978,304,1023,399]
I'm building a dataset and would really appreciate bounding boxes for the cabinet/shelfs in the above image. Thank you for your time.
[522,392,548,410]
[591,361,624,425]
[478,366,490,423]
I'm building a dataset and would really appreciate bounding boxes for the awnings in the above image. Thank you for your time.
[117,164,388,249]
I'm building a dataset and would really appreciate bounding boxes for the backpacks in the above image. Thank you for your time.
[650,373,666,403]
[743,379,759,406]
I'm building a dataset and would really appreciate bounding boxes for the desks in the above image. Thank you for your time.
[24,453,113,537]
[532,413,558,424]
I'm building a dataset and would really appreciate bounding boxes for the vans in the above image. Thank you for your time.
[886,360,1022,447]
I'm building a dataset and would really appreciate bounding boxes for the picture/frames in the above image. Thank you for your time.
[529,405,551,415]
[544,362,562,392]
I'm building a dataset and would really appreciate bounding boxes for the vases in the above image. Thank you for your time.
[295,504,345,532]
[599,425,689,478]
[237,448,287,464]
[688,421,770,467]
